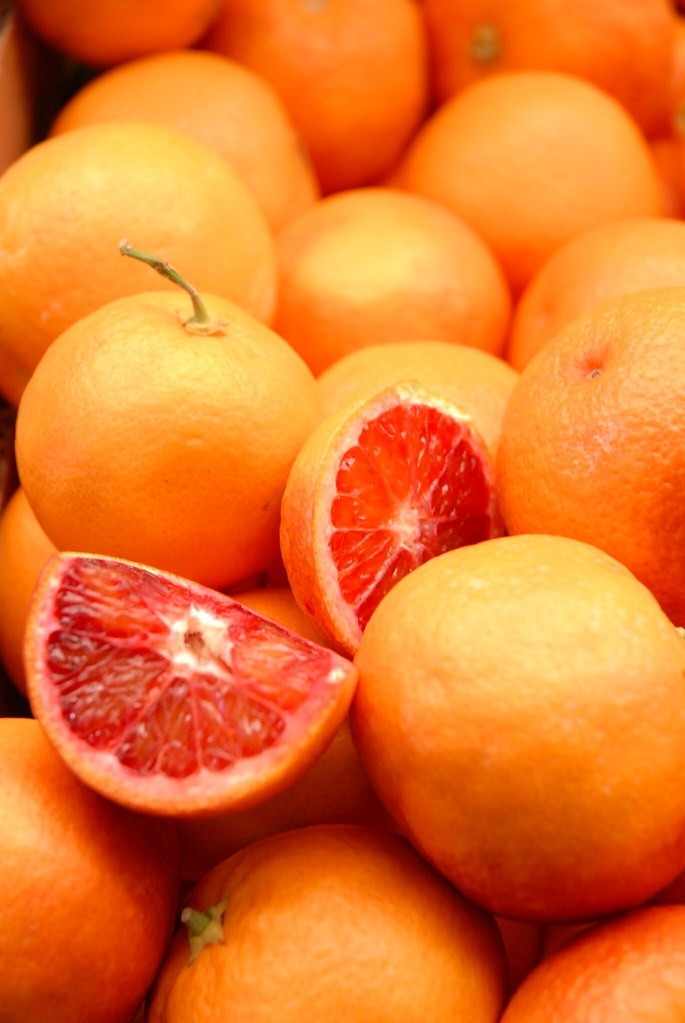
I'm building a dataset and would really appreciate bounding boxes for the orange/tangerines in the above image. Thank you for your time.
[0,0,684,1023]
